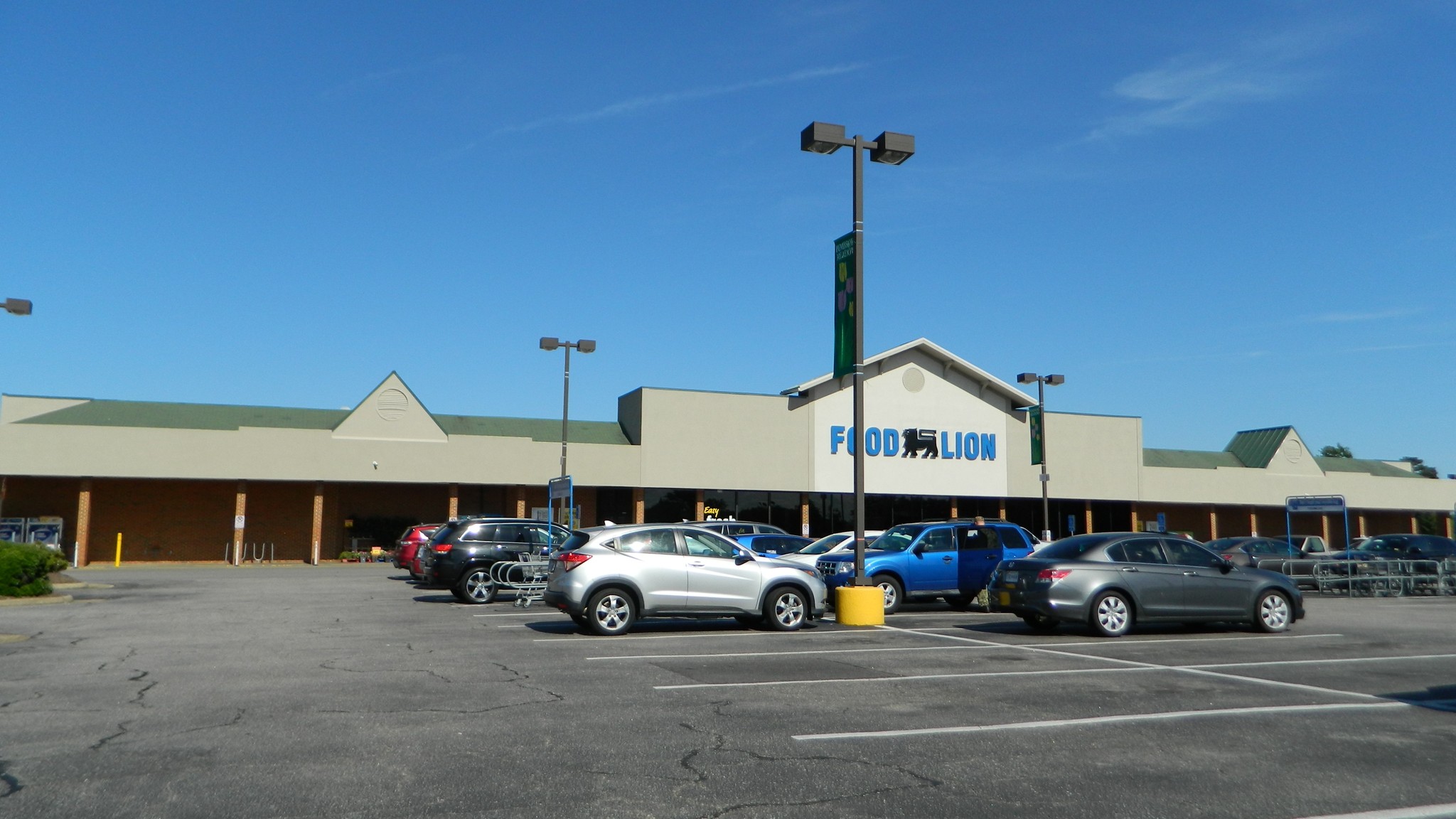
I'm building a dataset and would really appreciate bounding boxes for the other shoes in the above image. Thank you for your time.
[980,607,990,613]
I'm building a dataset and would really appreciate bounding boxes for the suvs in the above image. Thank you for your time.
[812,520,1035,616]
[414,517,572,605]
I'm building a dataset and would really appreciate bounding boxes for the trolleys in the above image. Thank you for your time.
[515,552,553,608]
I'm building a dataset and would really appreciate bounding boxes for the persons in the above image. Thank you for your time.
[621,534,651,552]
[972,517,998,612]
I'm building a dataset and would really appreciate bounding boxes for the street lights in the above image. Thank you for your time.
[1016,372,1064,542]
[799,122,917,590]
[538,336,596,526]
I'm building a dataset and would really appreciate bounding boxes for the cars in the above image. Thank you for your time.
[670,519,832,558]
[968,526,1057,555]
[987,531,1305,639]
[1275,533,1456,591]
[391,523,445,581]
[1200,536,1343,591]
[541,521,830,637]
[772,530,936,569]
